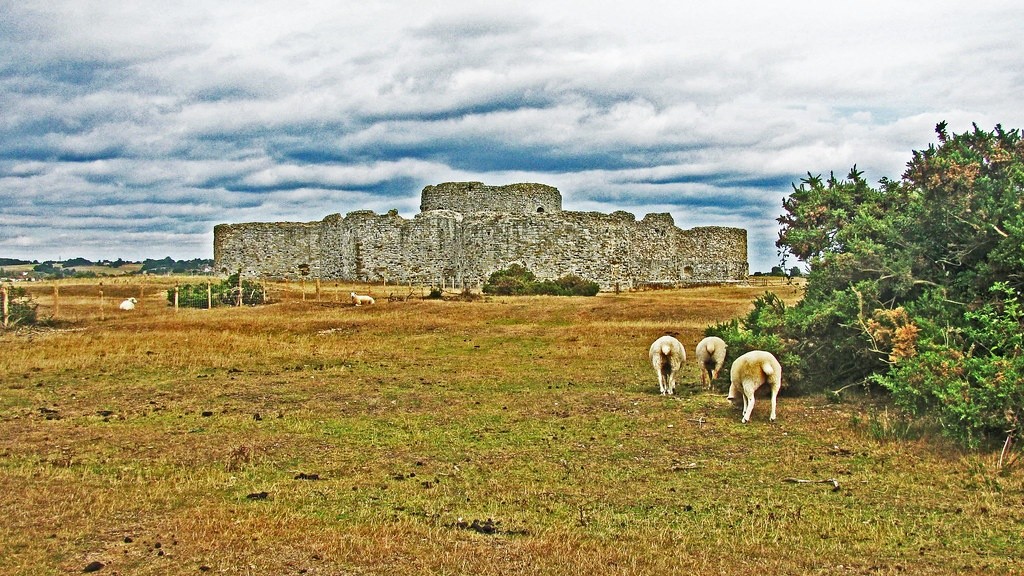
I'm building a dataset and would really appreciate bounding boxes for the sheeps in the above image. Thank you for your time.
[119,297,137,310]
[349,292,375,306]
[648,334,782,424]
